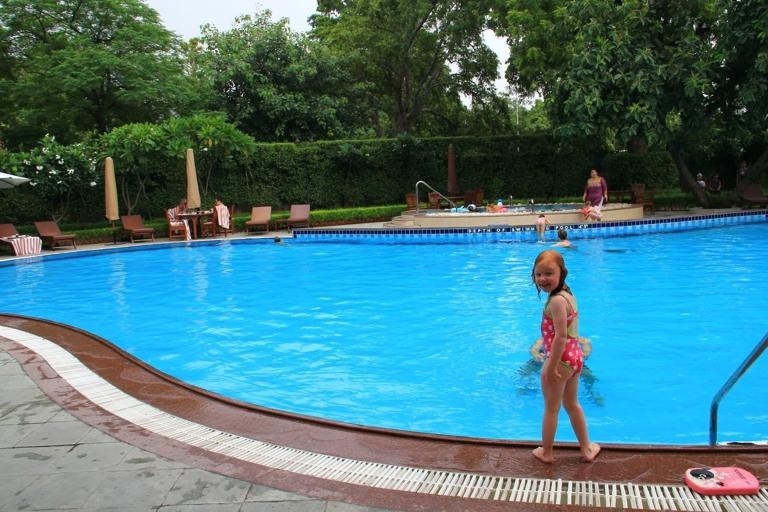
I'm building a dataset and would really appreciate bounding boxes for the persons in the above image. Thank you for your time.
[582,168,608,213]
[551,229,572,248]
[207,196,231,230]
[584,201,600,223]
[519,335,603,408]
[485,199,509,213]
[536,214,553,239]
[174,199,201,238]
[529,249,601,464]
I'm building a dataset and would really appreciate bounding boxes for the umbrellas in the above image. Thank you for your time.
[104,157,127,245]
[447,144,457,208]
[185,149,201,239]
[0,170,32,190]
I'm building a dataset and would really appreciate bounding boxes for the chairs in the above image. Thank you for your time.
[631,182,657,215]
[273,202,312,235]
[404,189,418,210]
[34,220,79,251]
[120,214,156,244]
[244,204,272,235]
[166,198,237,241]
[0,223,43,258]
[426,190,441,209]
